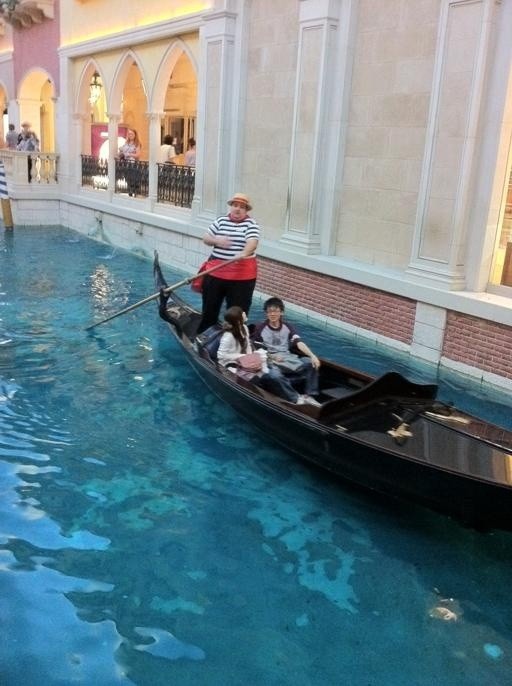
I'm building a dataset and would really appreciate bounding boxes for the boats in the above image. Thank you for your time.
[154,250,512,532]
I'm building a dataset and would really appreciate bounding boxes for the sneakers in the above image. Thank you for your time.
[304,396,320,407]
[295,394,304,404]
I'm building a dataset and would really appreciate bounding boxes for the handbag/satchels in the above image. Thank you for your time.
[237,352,262,372]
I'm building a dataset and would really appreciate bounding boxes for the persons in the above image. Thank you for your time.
[119,128,143,196]
[214,304,266,390]
[194,191,261,335]
[158,134,178,168]
[184,137,197,167]
[5,120,40,182]
[250,295,326,408]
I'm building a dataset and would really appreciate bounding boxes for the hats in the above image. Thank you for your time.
[227,193,252,211]
